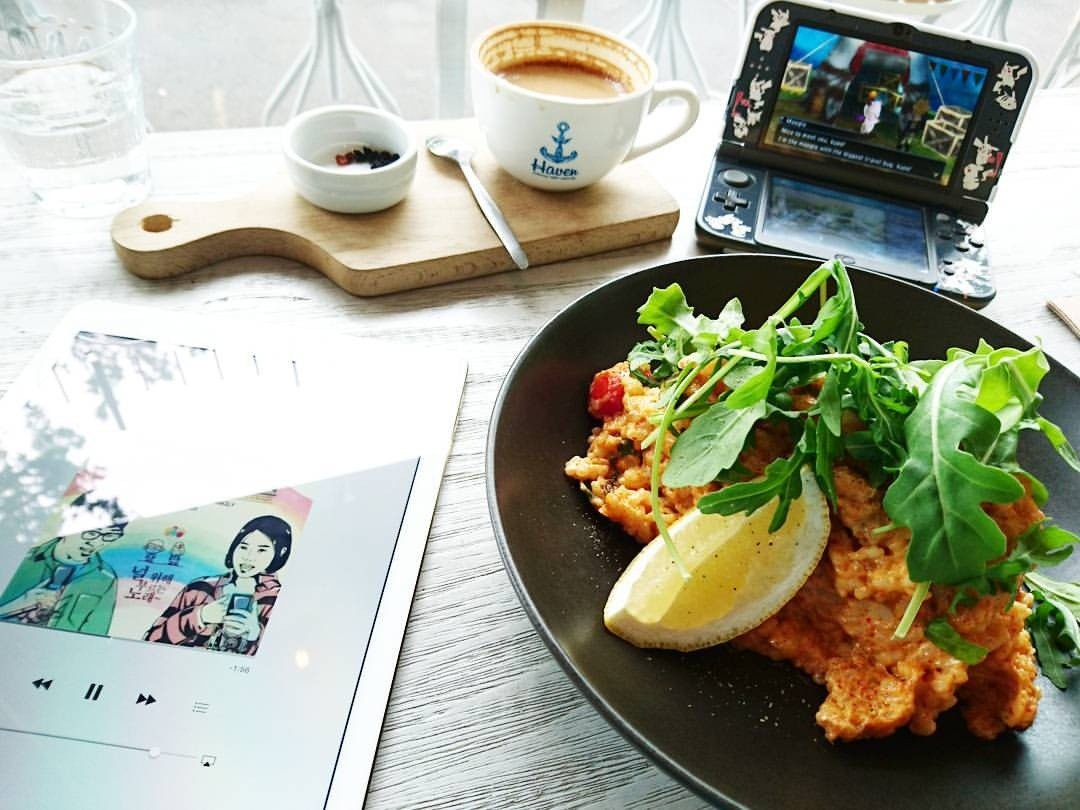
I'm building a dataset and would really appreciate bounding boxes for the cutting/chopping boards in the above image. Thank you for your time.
[110,121,681,296]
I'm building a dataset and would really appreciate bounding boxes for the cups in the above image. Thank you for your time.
[470,17,700,191]
[0,1,153,217]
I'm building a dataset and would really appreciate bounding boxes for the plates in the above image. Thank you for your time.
[484,253,1080,810]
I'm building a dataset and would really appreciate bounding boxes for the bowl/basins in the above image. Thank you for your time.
[286,104,418,213]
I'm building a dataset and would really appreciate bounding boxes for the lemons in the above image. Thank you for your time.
[603,462,831,650]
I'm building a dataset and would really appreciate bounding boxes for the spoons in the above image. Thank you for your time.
[424,133,531,271]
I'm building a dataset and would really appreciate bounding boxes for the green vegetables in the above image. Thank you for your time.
[622,257,1080,690]
